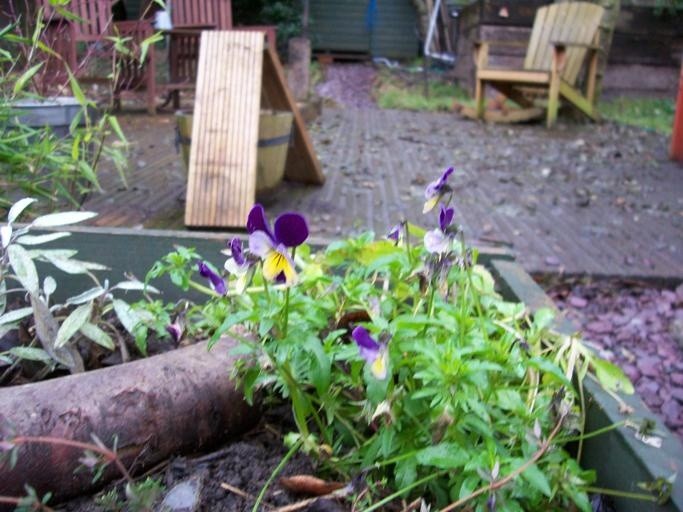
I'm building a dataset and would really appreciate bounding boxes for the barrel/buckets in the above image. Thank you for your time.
[2,97,96,206]
[174,108,293,207]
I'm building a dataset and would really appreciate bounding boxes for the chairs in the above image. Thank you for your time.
[468,0,603,131]
[37,0,157,116]
[169,0,277,108]
